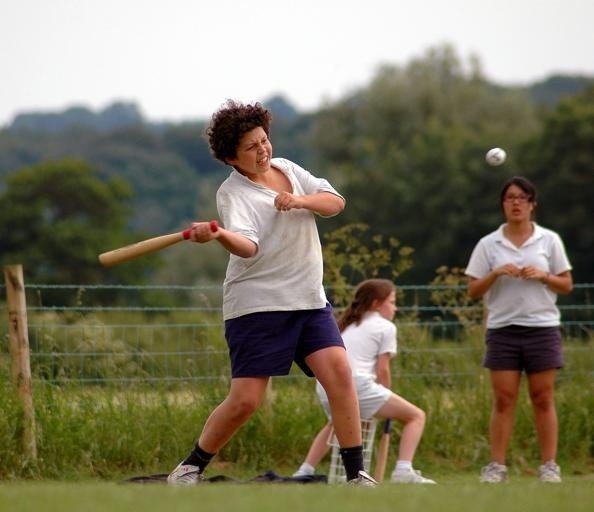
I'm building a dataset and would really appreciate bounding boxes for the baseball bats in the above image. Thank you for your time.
[374,419,390,482]
[98,220,218,268]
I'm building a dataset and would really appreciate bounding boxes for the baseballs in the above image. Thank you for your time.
[486,147,506,166]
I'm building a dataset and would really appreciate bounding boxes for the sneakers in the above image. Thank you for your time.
[476,462,509,485]
[536,459,562,484]
[166,455,203,489]
[389,466,439,486]
[343,470,381,486]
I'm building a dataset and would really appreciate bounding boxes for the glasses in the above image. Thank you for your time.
[502,193,534,203]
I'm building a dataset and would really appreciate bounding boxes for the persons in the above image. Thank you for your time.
[466,175,574,484]
[166,99,381,488]
[292,278,439,486]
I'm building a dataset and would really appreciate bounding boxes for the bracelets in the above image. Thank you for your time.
[542,272,550,285]
